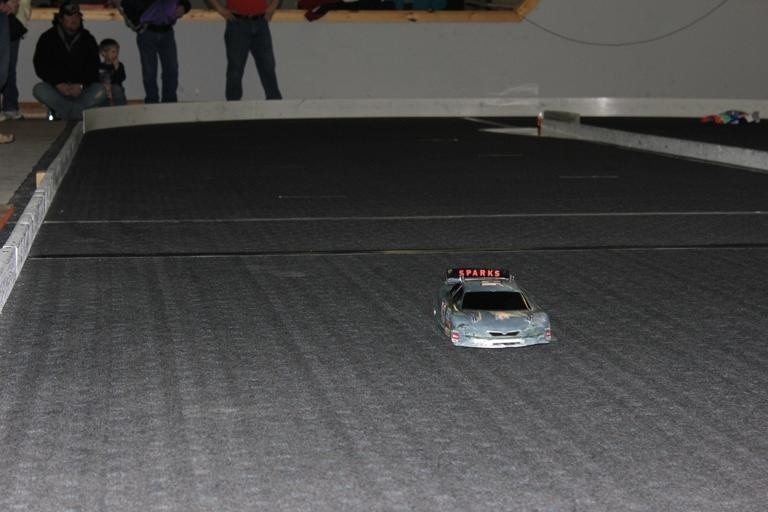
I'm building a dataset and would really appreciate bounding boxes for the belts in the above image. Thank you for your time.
[230,11,267,21]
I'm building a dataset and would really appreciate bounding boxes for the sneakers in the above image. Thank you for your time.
[0,108,23,144]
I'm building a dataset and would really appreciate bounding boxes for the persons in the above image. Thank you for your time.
[0,1,32,124]
[32,1,109,124]
[208,0,283,102]
[116,1,195,104]
[0,0,19,146]
[94,37,128,106]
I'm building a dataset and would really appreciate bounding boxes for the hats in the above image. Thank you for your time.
[60,2,84,17]
